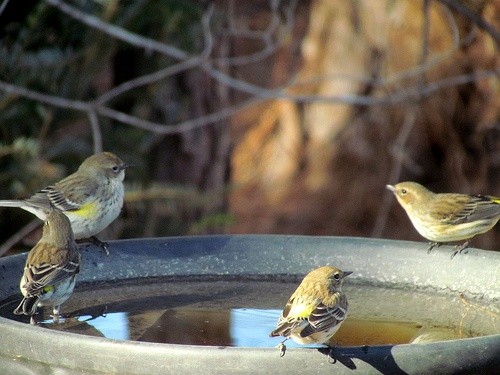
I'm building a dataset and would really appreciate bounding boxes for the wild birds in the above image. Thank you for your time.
[385,181,500,260]
[14,201,81,325]
[268,265,354,364]
[0,153,135,255]
